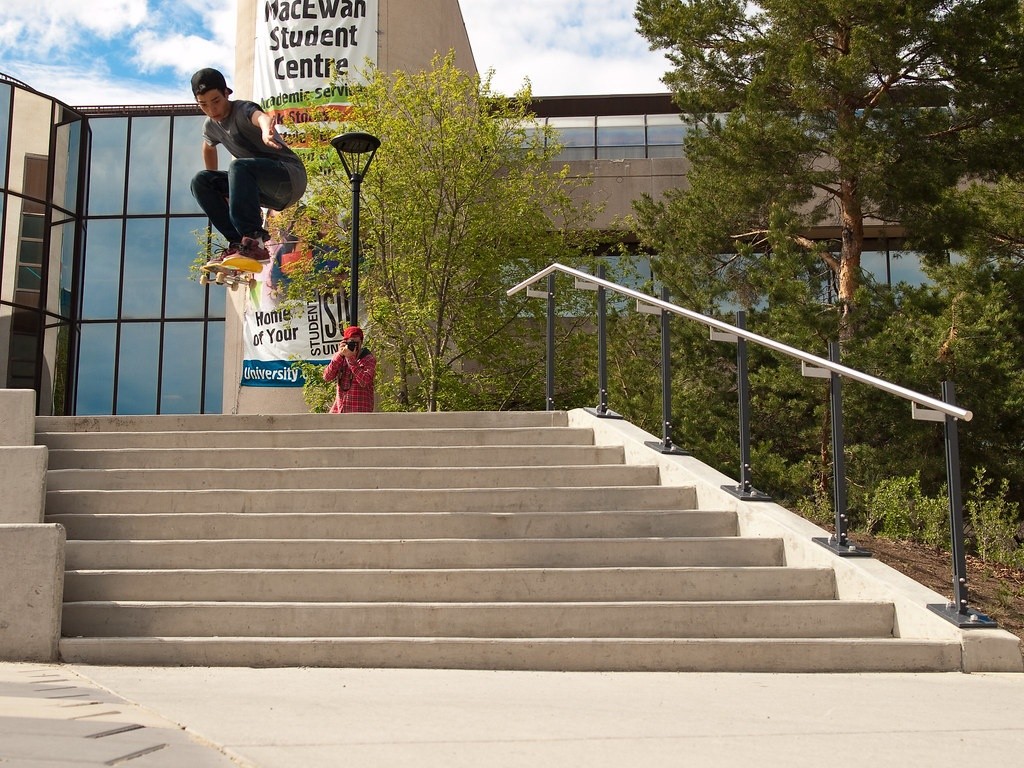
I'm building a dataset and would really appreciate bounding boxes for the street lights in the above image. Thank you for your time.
[330,132,383,332]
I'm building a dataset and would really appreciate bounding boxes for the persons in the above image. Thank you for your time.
[190,69,308,263]
[260,199,371,298]
[323,327,377,412]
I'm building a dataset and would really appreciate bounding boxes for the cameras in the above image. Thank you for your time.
[342,339,359,351]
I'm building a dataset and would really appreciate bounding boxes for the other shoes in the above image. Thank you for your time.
[206,242,243,263]
[221,237,272,264]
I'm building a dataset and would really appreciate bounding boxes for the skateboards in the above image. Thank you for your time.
[199,263,258,292]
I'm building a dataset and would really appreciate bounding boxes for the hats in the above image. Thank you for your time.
[342,326,363,340]
[191,67,234,101]
